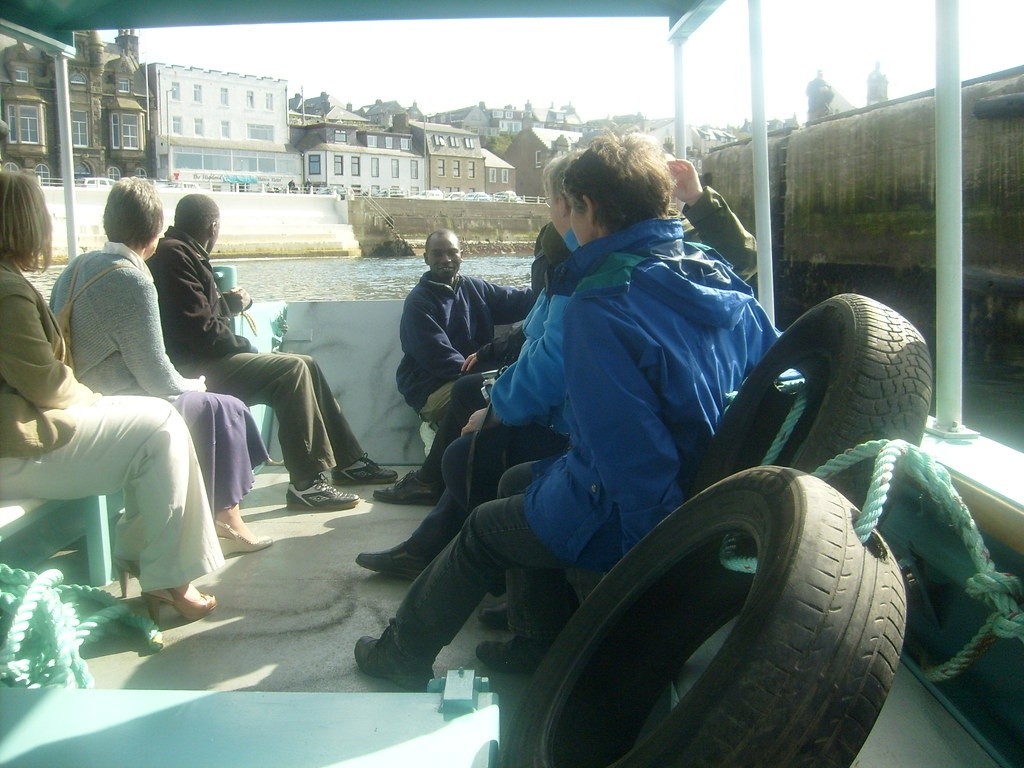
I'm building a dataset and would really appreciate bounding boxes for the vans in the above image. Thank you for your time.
[75,177,115,189]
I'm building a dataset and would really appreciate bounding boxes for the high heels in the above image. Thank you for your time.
[112,556,141,598]
[140,585,220,625]
[213,519,274,557]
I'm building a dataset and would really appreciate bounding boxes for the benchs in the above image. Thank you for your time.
[0,491,125,586]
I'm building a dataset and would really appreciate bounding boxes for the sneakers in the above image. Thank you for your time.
[330,457,400,485]
[285,475,361,511]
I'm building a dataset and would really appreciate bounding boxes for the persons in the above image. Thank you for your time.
[49,177,272,557]
[288,179,295,192]
[1,172,225,628]
[146,192,398,510]
[353,123,778,692]
[304,177,312,190]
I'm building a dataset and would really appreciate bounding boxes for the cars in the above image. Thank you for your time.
[492,191,516,202]
[408,190,444,200]
[466,192,489,201]
[445,192,465,200]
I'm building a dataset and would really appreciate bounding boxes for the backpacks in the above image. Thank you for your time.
[56,253,142,374]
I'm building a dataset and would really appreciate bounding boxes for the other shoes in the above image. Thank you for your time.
[475,635,550,674]
[477,577,581,629]
[353,617,435,693]
[481,568,508,598]
[355,541,425,581]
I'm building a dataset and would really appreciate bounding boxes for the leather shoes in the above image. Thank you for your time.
[372,470,440,505]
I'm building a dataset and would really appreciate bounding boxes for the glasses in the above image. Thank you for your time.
[544,197,553,208]
